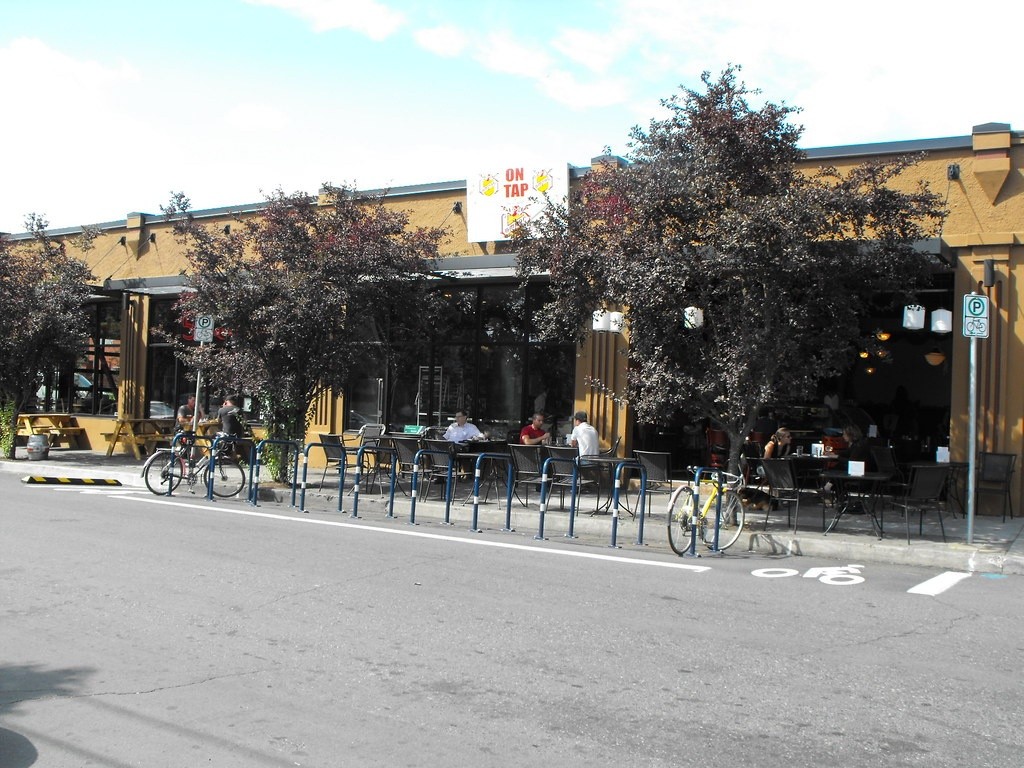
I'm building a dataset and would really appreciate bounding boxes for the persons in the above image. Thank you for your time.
[519,414,554,492]
[433,408,485,484]
[570,412,600,494]
[813,424,872,506]
[173,394,246,464]
[763,426,792,506]
[533,386,549,418]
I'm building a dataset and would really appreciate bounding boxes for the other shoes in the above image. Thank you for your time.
[815,486,833,493]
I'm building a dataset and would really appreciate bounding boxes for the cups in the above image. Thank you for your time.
[547,436,552,445]
[187,415,191,422]
[556,436,562,446]
[816,447,822,459]
[797,446,803,458]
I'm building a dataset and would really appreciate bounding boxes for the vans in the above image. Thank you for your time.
[37,370,94,411]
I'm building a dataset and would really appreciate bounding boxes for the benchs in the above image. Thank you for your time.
[101,430,253,449]
[16,426,84,437]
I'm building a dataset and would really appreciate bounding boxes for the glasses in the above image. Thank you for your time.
[785,435,791,438]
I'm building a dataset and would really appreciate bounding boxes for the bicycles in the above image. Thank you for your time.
[665,465,745,555]
[141,430,245,496]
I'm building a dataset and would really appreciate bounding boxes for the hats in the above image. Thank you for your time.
[573,411,588,421]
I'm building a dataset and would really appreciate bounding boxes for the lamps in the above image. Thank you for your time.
[684,306,703,328]
[902,305,953,334]
[592,300,627,335]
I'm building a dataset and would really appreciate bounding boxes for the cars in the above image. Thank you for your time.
[349,410,377,428]
[149,401,174,419]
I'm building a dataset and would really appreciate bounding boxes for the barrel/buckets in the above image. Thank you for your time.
[27,434,50,461]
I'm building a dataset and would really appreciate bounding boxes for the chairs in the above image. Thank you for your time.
[318,424,1016,546]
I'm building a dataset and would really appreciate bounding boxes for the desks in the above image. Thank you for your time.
[180,420,221,438]
[459,438,526,510]
[581,457,638,518]
[17,414,80,448]
[783,455,837,495]
[105,419,177,462]
